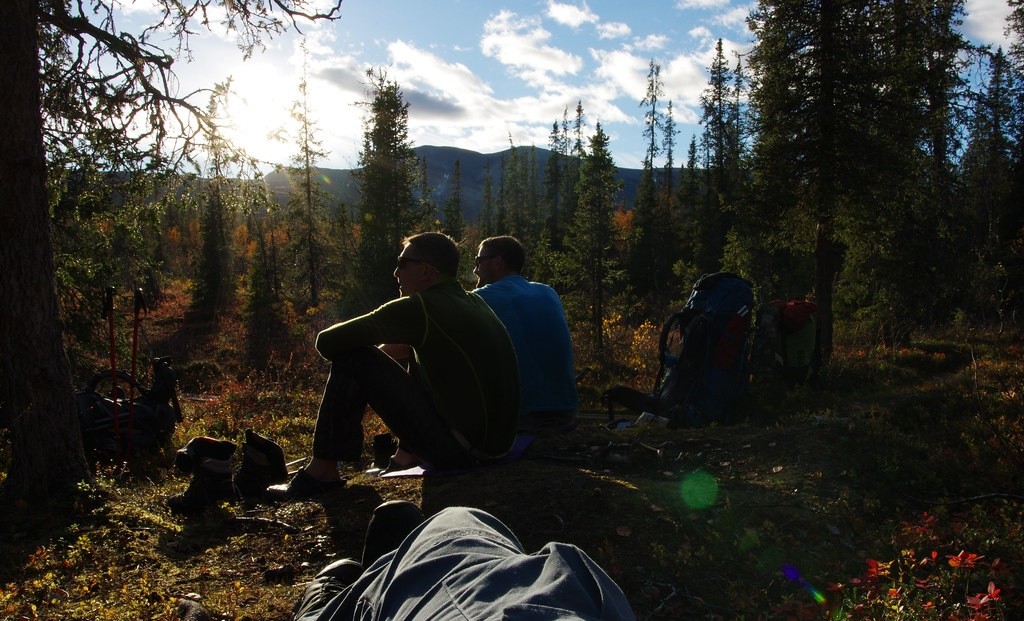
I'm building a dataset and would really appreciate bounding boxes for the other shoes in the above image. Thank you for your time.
[284,468,347,496]
[378,456,427,478]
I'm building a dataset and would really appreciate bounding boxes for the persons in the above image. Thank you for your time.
[262,231,520,504]
[292,500,635,621]
[467,236,577,428]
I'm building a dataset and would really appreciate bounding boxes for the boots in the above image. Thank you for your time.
[363,499,423,573]
[167,437,236,514]
[293,559,363,621]
[233,428,288,498]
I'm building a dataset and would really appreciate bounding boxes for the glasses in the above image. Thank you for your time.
[396,256,440,273]
[474,254,505,267]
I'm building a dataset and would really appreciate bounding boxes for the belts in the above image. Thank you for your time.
[450,426,516,462]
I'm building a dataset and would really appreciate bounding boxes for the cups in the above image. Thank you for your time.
[373,434,393,468]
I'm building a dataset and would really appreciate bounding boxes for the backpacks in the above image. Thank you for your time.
[72,354,180,454]
[654,269,754,422]
[746,300,817,387]
